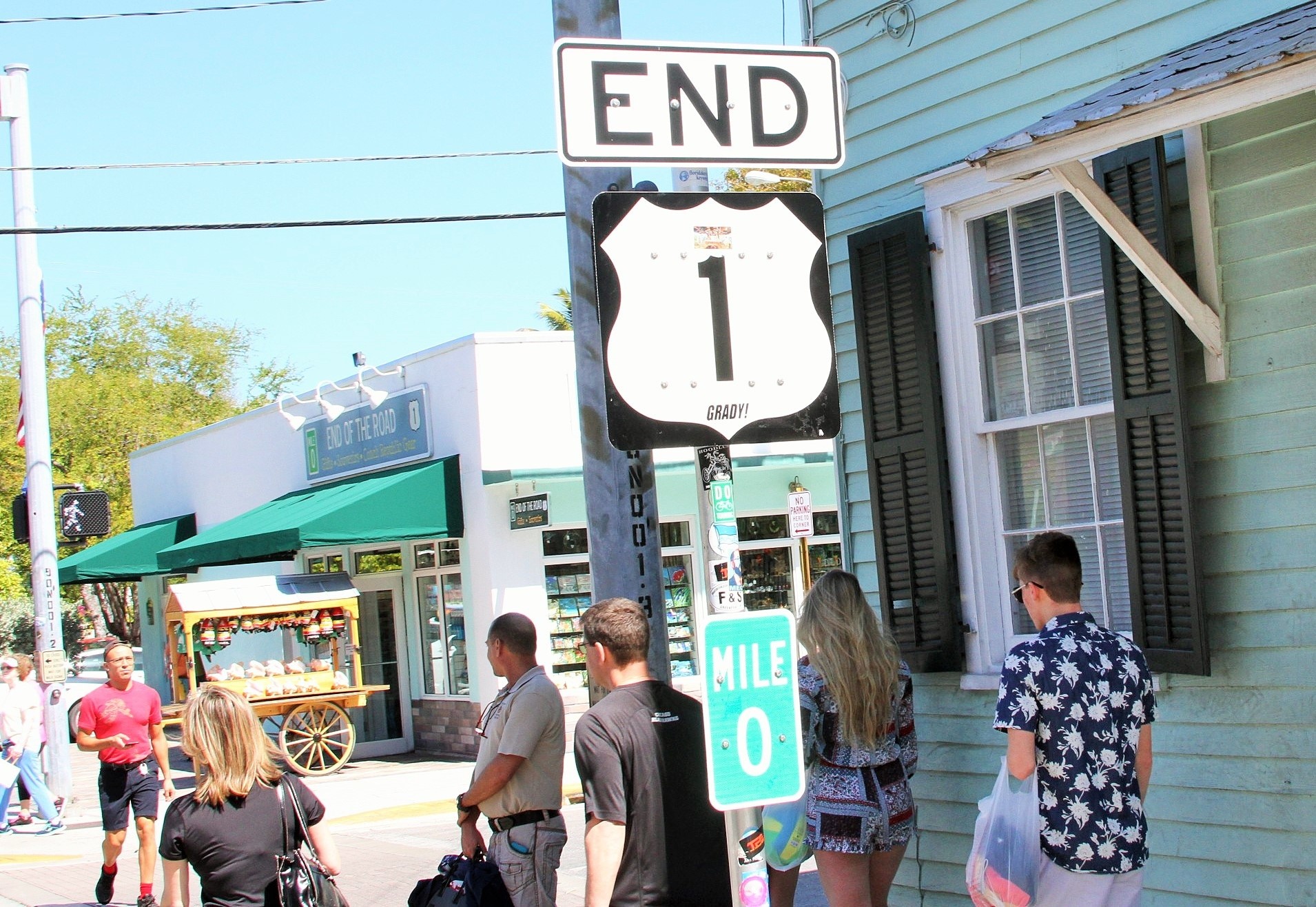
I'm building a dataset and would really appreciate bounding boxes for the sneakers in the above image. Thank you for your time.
[7,813,33,826]
[0,825,13,836]
[55,797,67,817]
[35,821,68,836]
[137,894,160,907]
[95,863,118,905]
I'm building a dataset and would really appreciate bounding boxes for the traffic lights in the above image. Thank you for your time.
[60,491,109,537]
[9,497,31,544]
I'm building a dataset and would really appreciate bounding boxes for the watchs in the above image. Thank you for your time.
[456,793,472,813]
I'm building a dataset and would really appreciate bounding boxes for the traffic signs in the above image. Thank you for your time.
[42,650,68,684]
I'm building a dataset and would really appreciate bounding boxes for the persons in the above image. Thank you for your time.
[456,612,567,907]
[766,860,800,907]
[574,598,733,907]
[158,682,350,907]
[0,653,70,839]
[797,569,918,907]
[76,640,175,907]
[965,532,1157,907]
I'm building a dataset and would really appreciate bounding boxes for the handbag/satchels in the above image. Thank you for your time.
[0,755,21,789]
[966,752,1042,907]
[762,761,815,872]
[408,846,513,907]
[275,774,349,907]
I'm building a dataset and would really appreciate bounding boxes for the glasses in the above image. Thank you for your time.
[578,642,603,655]
[109,658,135,665]
[1012,582,1084,603]
[474,700,501,739]
[0,667,13,670]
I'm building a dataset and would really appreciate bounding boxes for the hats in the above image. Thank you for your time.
[104,640,131,678]
[0,657,18,667]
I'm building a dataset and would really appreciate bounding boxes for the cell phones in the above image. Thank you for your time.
[511,842,529,854]
[126,741,140,745]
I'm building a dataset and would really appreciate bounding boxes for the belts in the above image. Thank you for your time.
[488,808,561,833]
[101,754,154,771]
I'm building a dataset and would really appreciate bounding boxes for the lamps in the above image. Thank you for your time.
[315,381,361,423]
[358,365,406,410]
[279,393,320,430]
[789,476,808,492]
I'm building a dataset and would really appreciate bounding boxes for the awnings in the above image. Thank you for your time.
[154,452,467,570]
[29,513,196,586]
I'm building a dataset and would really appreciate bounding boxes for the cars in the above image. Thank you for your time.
[61,647,145,738]
